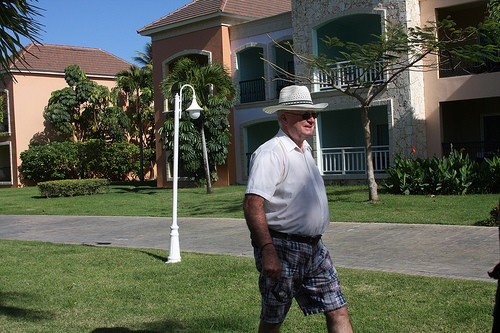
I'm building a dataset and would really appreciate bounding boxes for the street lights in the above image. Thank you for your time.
[166,84,205,264]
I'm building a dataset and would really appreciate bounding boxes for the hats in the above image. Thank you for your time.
[262,85,329,115]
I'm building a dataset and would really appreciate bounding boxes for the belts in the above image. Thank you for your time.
[268,228,322,248]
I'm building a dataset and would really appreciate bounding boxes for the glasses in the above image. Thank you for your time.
[284,112,319,120]
